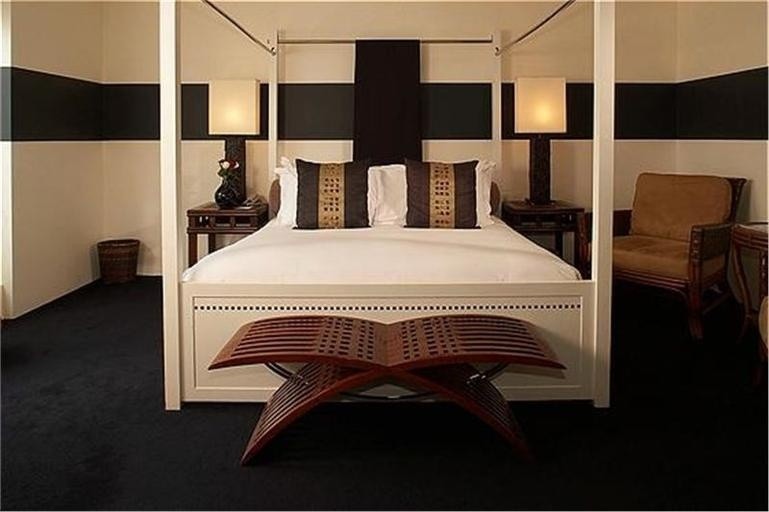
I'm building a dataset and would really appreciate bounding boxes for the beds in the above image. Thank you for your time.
[158,0,615,412]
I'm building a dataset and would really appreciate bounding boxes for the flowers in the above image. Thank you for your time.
[216,158,240,187]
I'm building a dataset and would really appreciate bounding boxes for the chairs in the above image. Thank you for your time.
[586,174,747,343]
[758,297,768,360]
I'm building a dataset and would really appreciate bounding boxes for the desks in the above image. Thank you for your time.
[733,221,768,334]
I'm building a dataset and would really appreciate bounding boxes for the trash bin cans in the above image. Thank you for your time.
[97,239,140,283]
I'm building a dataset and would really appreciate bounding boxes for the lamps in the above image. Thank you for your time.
[208,80,261,206]
[514,77,568,211]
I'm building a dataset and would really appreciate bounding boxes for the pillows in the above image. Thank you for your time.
[403,158,481,229]
[275,156,381,227]
[292,158,372,229]
[373,159,497,228]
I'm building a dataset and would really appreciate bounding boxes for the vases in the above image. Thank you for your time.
[214,177,239,209]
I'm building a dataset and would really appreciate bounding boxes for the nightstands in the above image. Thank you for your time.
[186,202,268,267]
[502,199,589,279]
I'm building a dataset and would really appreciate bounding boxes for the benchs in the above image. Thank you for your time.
[208,314,566,466]
